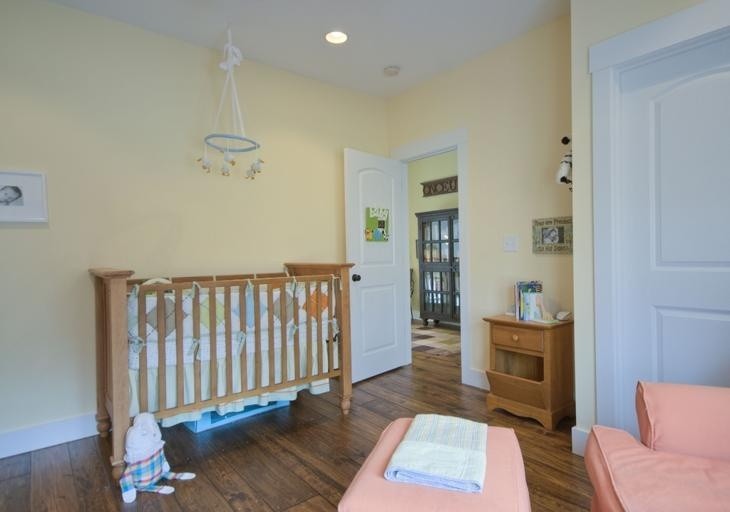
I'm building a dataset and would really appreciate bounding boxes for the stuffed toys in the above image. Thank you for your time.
[118,412,197,504]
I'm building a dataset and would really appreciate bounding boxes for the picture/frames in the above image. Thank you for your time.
[0,171,49,224]
[531,217,572,255]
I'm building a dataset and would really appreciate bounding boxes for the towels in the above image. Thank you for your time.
[384,413,487,495]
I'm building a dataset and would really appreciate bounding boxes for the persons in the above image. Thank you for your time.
[0,186,22,207]
[542,227,560,243]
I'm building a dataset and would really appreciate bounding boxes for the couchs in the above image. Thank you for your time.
[583,381,730,512]
[337,418,534,512]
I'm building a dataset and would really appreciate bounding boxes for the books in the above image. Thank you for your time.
[505,278,544,321]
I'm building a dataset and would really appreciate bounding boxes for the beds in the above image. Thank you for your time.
[87,262,358,488]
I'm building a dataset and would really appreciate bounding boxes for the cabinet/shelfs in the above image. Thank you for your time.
[483,312,574,430]
[414,207,460,327]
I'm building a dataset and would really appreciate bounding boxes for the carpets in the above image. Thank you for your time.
[411,324,461,357]
